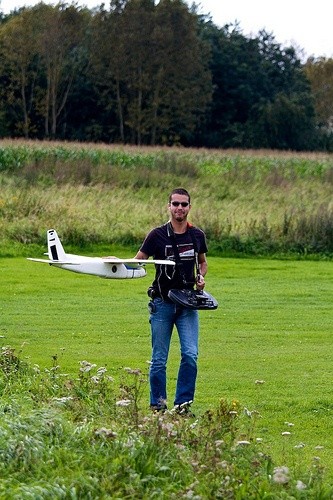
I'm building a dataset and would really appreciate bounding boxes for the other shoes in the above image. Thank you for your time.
[151,406,167,416]
[175,408,195,418]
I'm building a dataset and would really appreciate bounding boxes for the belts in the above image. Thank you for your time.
[155,292,172,301]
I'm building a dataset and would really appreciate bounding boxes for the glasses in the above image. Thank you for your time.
[170,202,190,207]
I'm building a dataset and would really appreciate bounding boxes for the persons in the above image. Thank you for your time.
[103,188,210,424]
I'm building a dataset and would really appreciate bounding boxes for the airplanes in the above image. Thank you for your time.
[26,229,176,279]
